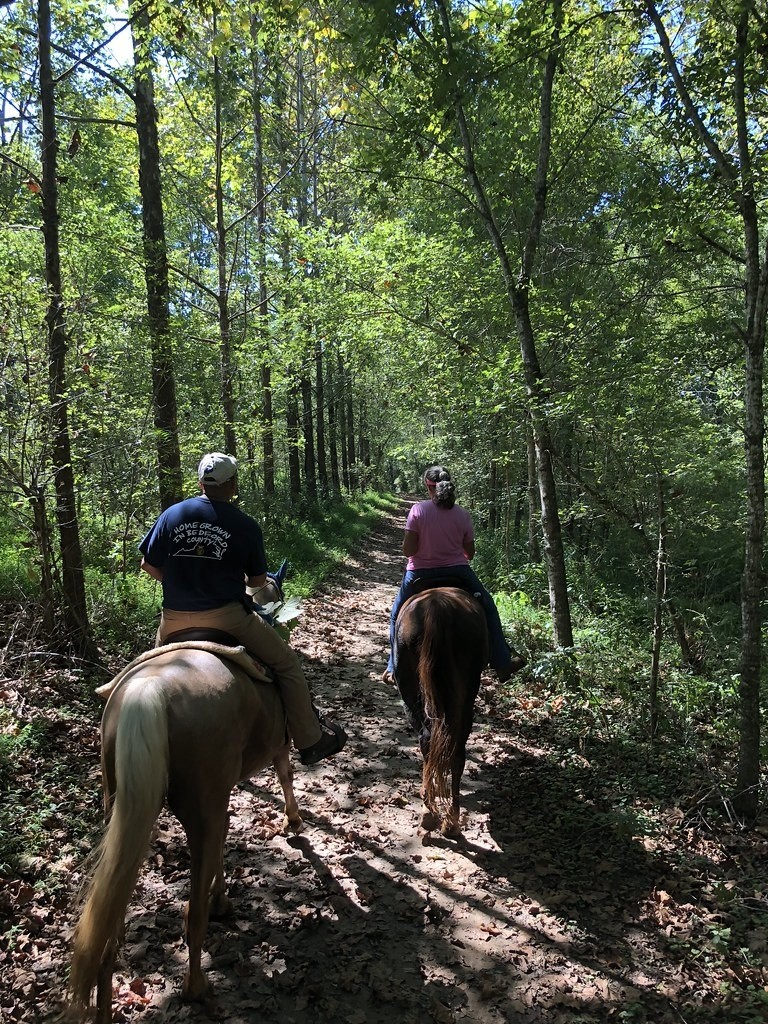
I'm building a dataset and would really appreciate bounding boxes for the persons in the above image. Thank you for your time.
[382,466,526,682]
[137,453,348,764]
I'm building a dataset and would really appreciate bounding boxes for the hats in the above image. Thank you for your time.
[198,452,238,485]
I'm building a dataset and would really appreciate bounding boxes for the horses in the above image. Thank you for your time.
[61,562,307,1024]
[389,587,485,843]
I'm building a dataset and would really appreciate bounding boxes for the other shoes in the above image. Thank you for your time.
[383,671,397,685]
[298,730,339,766]
[499,656,524,684]
